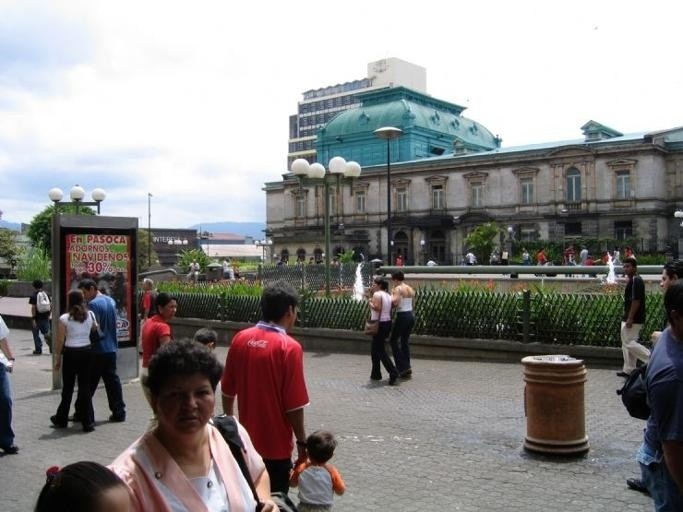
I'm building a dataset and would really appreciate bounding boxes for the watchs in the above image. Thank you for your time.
[296,439,307,448]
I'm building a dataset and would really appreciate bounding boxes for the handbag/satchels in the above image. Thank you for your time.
[255,490,299,512]
[364,320,379,335]
[621,363,652,420]
[90,325,104,342]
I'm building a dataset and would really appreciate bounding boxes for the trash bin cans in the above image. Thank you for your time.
[521,353,589,461]
[206,263,223,281]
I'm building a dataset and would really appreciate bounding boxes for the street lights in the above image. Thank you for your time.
[254,240,273,264]
[50,185,104,217]
[290,153,360,297]
[371,124,405,261]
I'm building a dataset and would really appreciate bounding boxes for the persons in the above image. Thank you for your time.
[221,257,235,280]
[290,429,344,511]
[184,259,200,286]
[387,271,415,378]
[34,461,131,512]
[394,255,405,267]
[366,277,399,384]
[426,258,438,266]
[230,257,240,278]
[465,250,477,266]
[106,337,279,512]
[636,278,683,511]
[139,278,178,420]
[268,255,343,268]
[614,258,653,377]
[625,258,683,493]
[220,282,309,495]
[29,280,53,354]
[0,314,18,455]
[195,328,217,351]
[488,243,638,279]
[50,278,126,432]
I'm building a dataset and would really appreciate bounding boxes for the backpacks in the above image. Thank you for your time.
[36,290,51,313]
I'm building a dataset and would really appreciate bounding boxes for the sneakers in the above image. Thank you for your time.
[33,350,42,355]
[371,374,382,380]
[616,372,630,378]
[50,414,96,432]
[627,478,643,492]
[388,368,412,386]
[0,444,19,455]
[109,413,126,422]
